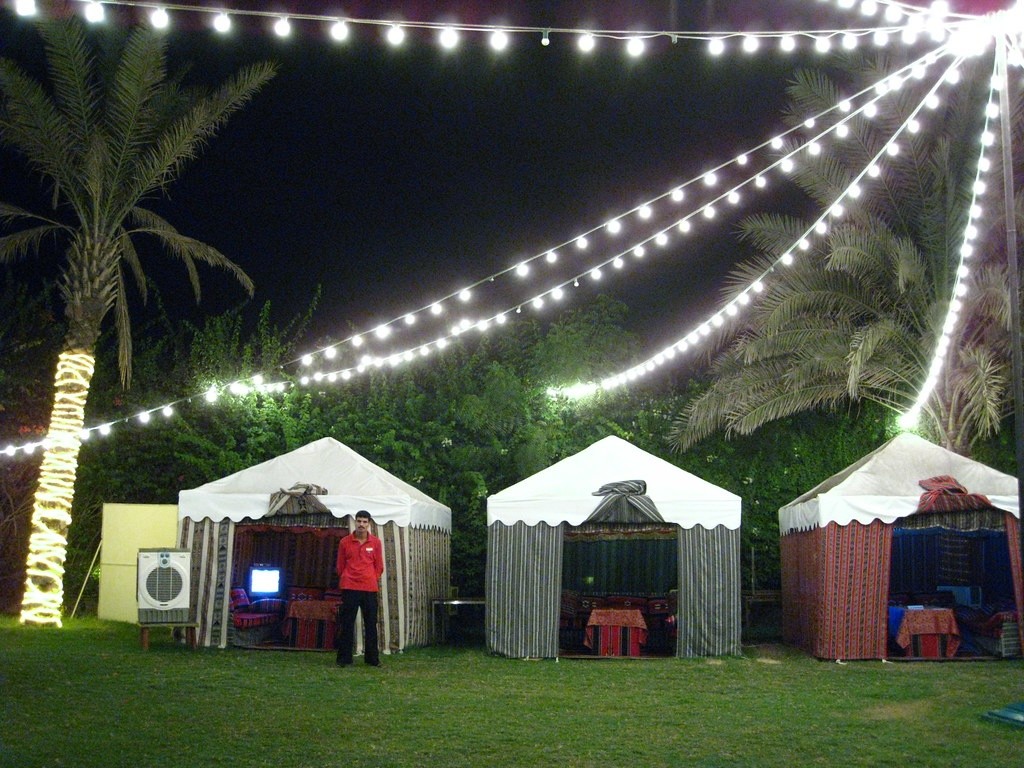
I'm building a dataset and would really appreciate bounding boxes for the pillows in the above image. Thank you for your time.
[560,590,584,616]
[231,586,250,609]
[249,598,287,613]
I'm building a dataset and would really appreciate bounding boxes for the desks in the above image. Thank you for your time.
[284,600,344,648]
[137,622,200,651]
[583,606,650,658]
[431,598,484,646]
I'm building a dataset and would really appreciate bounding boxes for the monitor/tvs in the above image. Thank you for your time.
[248,567,282,599]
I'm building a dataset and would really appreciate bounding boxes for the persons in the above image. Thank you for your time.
[336,509,383,667]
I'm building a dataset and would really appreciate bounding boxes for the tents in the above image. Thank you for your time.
[486,435,743,661]
[177,436,452,657]
[778,432,1024,661]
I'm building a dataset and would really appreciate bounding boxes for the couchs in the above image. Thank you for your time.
[958,601,1021,658]
[234,586,342,646]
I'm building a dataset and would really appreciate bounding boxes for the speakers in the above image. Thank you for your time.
[136,548,192,624]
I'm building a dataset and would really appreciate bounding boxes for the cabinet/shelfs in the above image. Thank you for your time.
[895,605,961,659]
